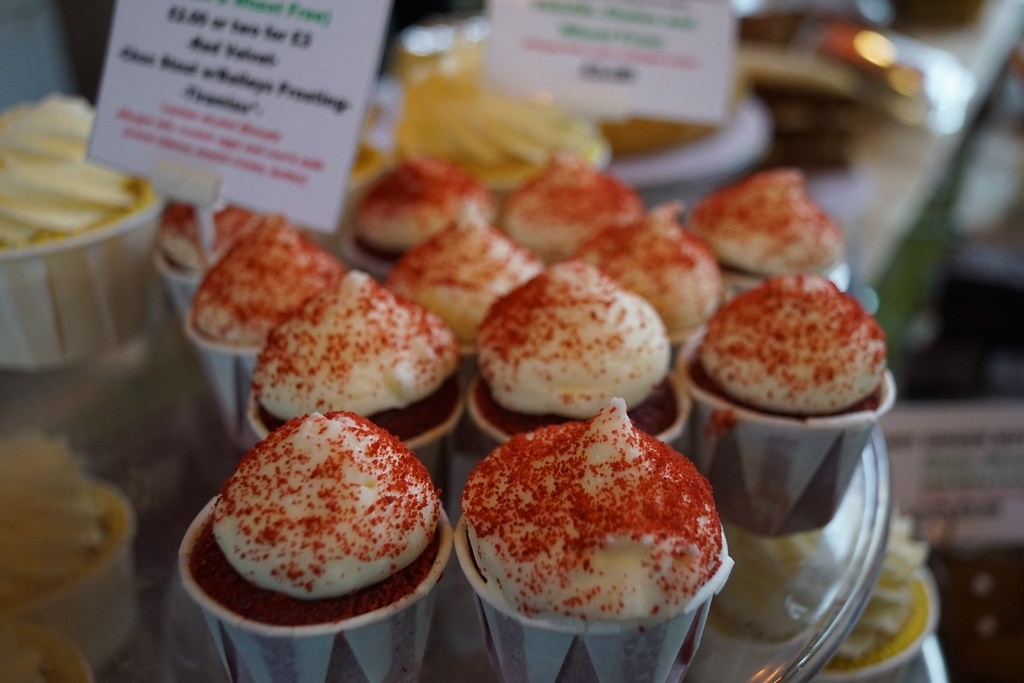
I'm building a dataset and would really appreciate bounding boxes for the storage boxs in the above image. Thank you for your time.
[881,245,1024,549]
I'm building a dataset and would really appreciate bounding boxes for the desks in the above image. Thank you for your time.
[778,1,1024,294]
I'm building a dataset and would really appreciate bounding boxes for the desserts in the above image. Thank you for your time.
[0,33,939,683]
